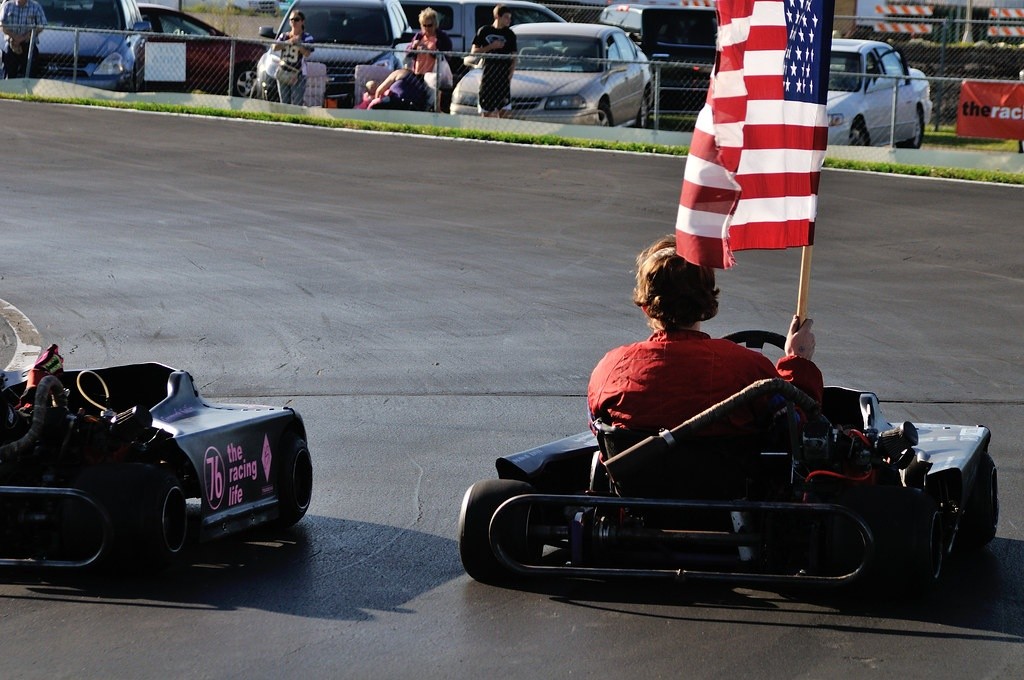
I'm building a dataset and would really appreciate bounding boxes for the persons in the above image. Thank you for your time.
[587,235,825,464]
[363,7,453,111]
[471,5,517,119]
[0,343,63,444]
[273,10,315,105]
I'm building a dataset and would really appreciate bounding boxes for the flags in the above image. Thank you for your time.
[675,0,836,270]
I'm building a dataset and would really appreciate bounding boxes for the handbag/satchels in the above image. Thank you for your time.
[274,61,299,85]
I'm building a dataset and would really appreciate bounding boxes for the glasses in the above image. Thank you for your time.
[291,17,300,22]
[423,24,433,28]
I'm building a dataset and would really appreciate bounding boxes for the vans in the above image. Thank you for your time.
[597,1,721,128]
[399,0,570,58]
[255,0,413,110]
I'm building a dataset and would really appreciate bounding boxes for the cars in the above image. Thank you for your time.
[0,0,152,94]
[447,23,657,128]
[137,2,269,100]
[828,36,936,150]
